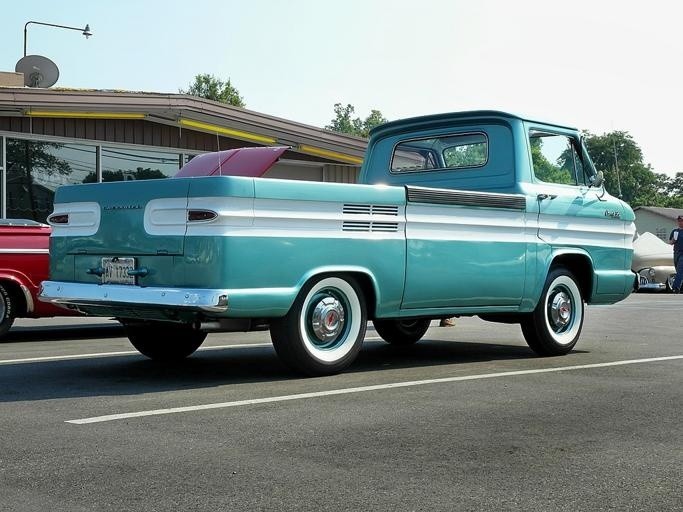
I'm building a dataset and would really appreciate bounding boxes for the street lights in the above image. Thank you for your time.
[24,20,93,58]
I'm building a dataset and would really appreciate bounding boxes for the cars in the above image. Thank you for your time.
[631,230,683,295]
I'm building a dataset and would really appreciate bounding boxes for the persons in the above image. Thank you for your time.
[439,318,457,327]
[668,214,683,294]
